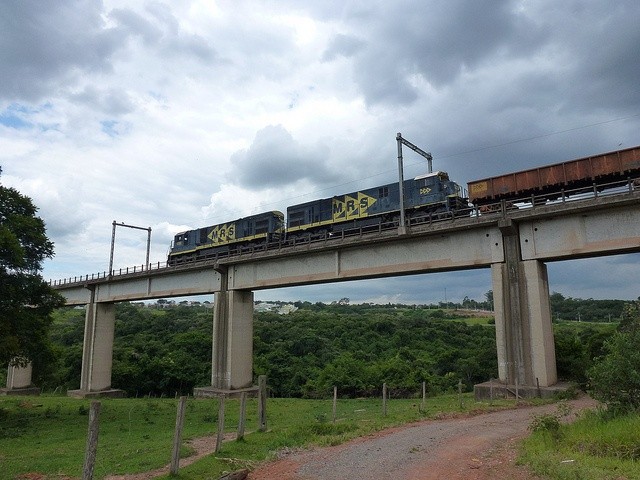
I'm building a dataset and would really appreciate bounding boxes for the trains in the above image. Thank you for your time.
[167,146,640,267]
